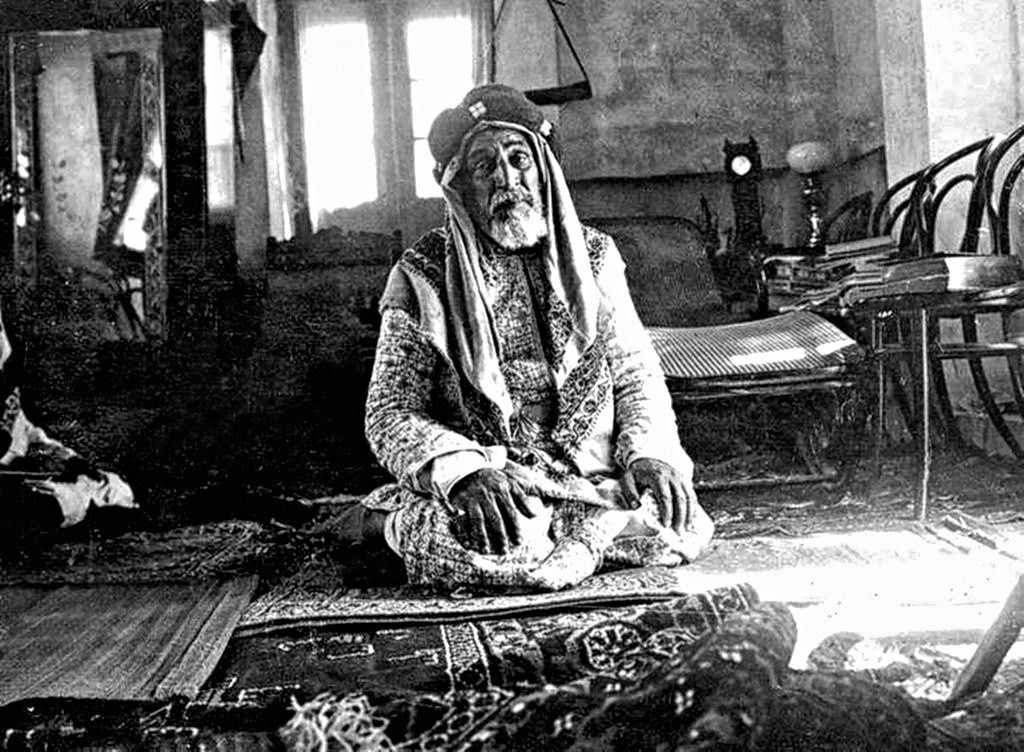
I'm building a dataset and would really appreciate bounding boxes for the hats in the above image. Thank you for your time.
[428,83,563,165]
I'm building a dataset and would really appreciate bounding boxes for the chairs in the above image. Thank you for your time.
[758,125,1024,519]
[581,213,866,493]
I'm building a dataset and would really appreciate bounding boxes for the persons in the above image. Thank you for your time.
[276,85,715,601]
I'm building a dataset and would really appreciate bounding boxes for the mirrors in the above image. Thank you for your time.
[0,0,205,370]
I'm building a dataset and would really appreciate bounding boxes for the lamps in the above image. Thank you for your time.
[787,136,834,247]
